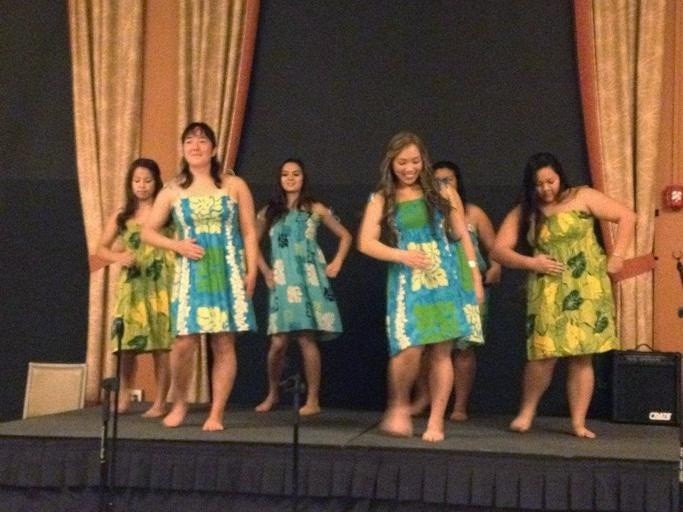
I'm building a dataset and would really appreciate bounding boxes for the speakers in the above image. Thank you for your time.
[604,350,681,426]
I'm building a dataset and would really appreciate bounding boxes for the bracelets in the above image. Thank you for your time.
[467,260,477,267]
[611,252,624,259]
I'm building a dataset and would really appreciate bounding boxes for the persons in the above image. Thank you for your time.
[248,159,354,417]
[490,152,640,439]
[356,132,485,443]
[140,124,259,432]
[98,158,180,422]
[402,157,502,423]
